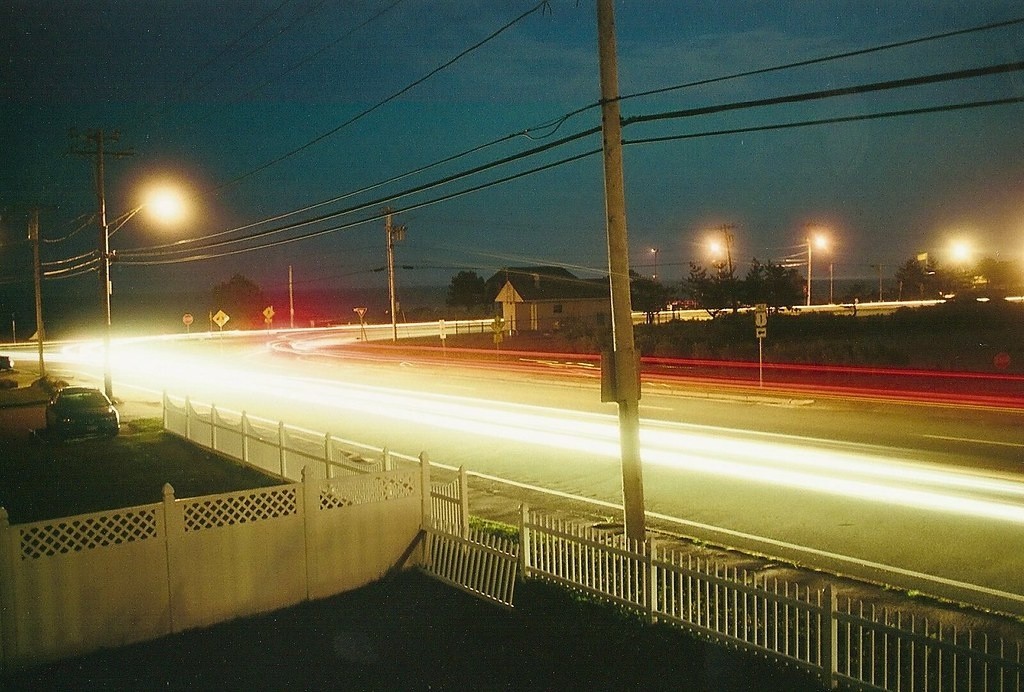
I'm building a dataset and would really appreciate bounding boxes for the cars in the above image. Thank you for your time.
[46,386,121,442]
[0,355,14,371]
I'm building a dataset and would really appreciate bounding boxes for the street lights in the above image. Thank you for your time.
[103,178,192,406]
[650,247,663,312]
[806,232,831,307]
[707,242,738,311]
